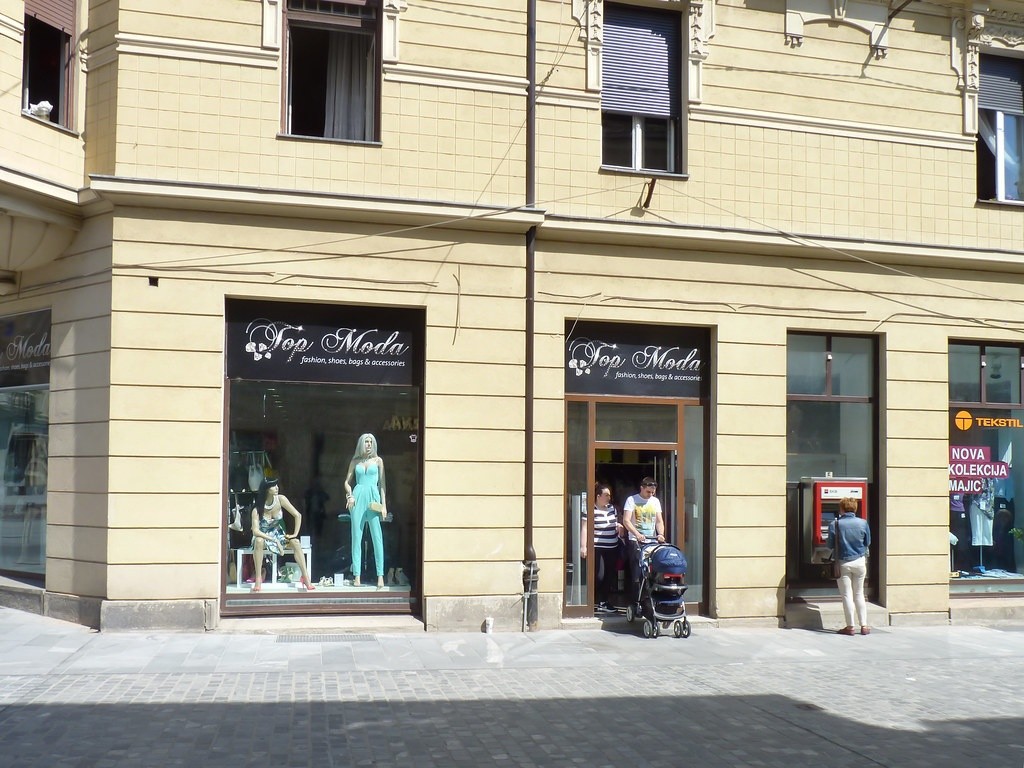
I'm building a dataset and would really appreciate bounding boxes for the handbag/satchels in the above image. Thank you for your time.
[229,451,302,583]
[833,562,841,578]
[369,501,383,512]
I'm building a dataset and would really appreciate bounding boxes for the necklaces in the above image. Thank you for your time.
[264,497,277,510]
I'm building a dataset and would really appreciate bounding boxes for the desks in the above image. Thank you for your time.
[237,548,313,589]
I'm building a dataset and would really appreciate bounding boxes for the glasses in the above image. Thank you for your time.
[642,482,658,487]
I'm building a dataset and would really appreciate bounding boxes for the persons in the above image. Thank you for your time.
[4,420,47,563]
[344,433,388,588]
[623,477,666,587]
[826,497,872,635]
[580,483,624,612]
[251,476,316,592]
[950,477,994,572]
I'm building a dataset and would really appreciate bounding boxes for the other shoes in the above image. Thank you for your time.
[861,627,870,635]
[318,575,334,586]
[837,627,854,635]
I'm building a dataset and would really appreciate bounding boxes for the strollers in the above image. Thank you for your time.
[625,535,691,640]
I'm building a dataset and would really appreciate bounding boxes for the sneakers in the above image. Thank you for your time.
[594,603,598,613]
[598,602,618,613]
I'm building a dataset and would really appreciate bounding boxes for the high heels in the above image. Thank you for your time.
[395,567,407,586]
[254,579,261,591]
[300,576,315,590]
[386,567,394,586]
